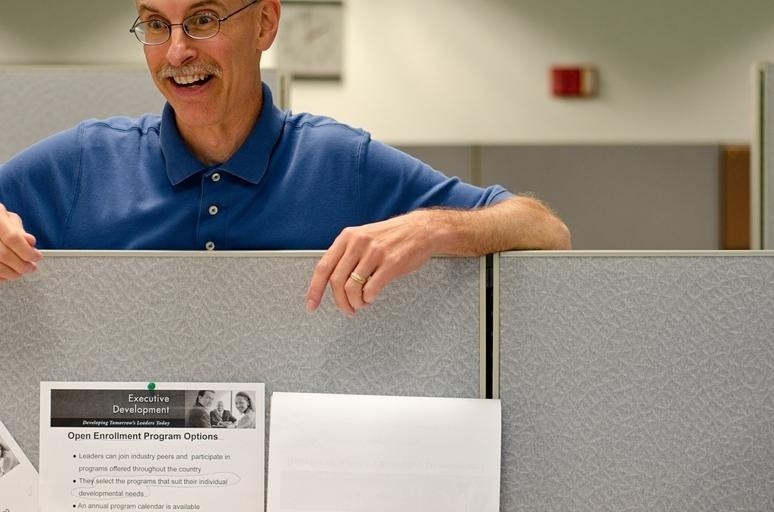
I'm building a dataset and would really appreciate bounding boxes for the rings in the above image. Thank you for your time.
[349,271,367,285]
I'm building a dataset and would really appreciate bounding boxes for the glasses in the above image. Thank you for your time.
[129,1,257,46]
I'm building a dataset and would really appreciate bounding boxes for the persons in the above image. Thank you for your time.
[210,401,237,428]
[227,391,256,428]
[0,1,573,319]
[189,390,216,428]
